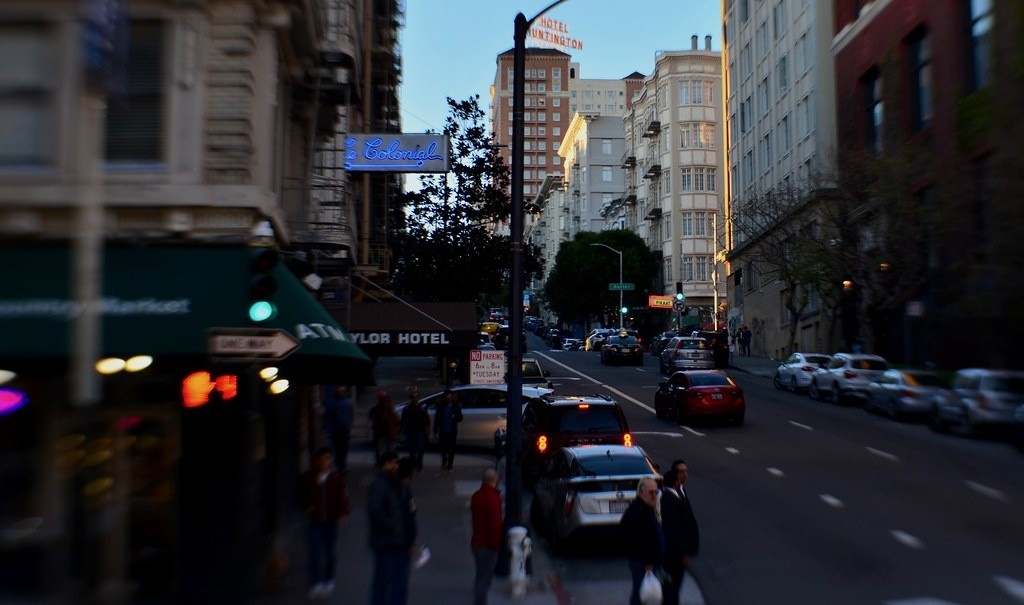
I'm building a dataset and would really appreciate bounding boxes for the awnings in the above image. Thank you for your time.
[350,301,477,332]
[0,235,372,388]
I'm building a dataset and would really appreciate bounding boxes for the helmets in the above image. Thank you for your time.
[376,390,388,399]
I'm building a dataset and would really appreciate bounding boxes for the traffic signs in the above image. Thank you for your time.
[208,326,303,363]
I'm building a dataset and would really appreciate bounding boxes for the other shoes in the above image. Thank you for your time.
[314,584,335,593]
[443,457,448,465]
[447,464,453,469]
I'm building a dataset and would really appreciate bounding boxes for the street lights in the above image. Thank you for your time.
[590,243,624,330]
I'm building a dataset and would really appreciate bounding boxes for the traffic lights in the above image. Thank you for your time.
[676,281,685,301]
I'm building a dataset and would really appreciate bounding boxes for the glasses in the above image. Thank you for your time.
[315,456,331,463]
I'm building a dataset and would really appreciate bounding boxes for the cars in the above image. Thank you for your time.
[654,368,746,426]
[772,351,835,392]
[866,368,946,419]
[473,307,645,383]
[384,384,555,452]
[527,441,668,544]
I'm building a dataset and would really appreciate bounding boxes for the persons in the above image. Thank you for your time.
[331,399,354,472]
[729,326,752,357]
[292,446,351,588]
[369,389,399,472]
[470,469,502,605]
[660,459,700,605]
[619,477,667,605]
[398,388,430,471]
[362,452,422,605]
[433,389,463,471]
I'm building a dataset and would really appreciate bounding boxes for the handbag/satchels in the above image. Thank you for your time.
[640,570,663,605]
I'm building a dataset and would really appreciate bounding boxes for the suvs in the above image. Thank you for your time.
[659,336,716,374]
[809,352,890,403]
[931,367,1024,435]
[518,392,634,472]
